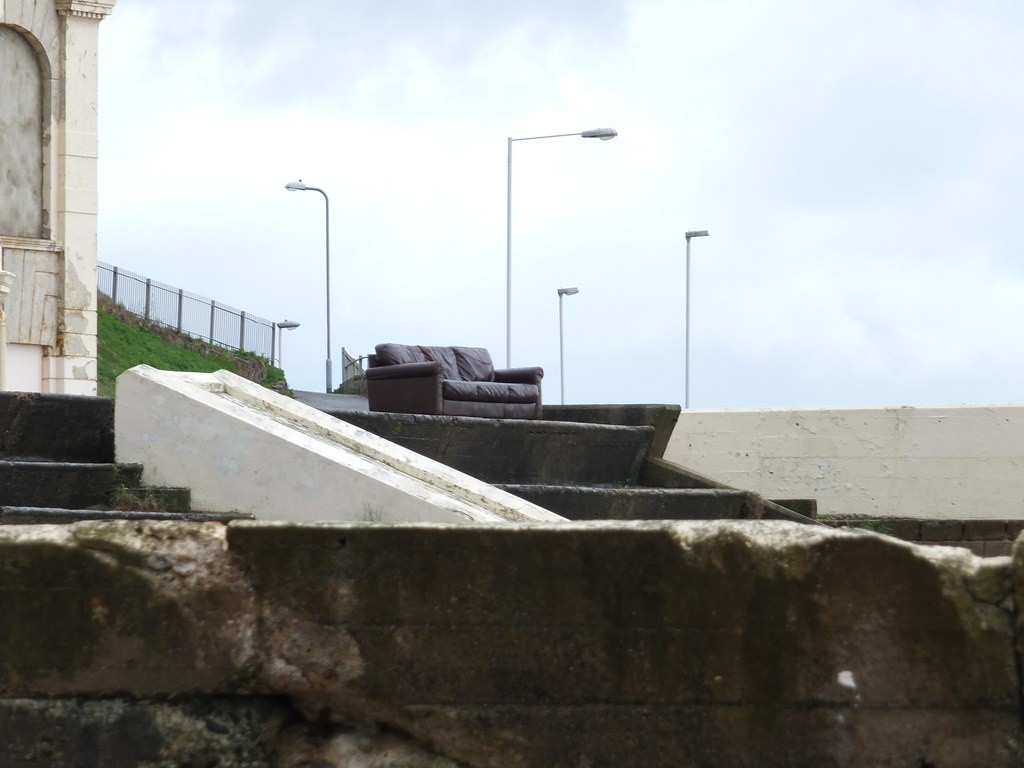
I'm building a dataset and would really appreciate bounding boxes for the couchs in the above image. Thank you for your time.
[366,341,544,421]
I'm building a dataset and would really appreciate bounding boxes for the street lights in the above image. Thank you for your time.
[555,285,583,405]
[277,319,302,373]
[505,127,618,366]
[286,179,335,393]
[683,231,710,407]
